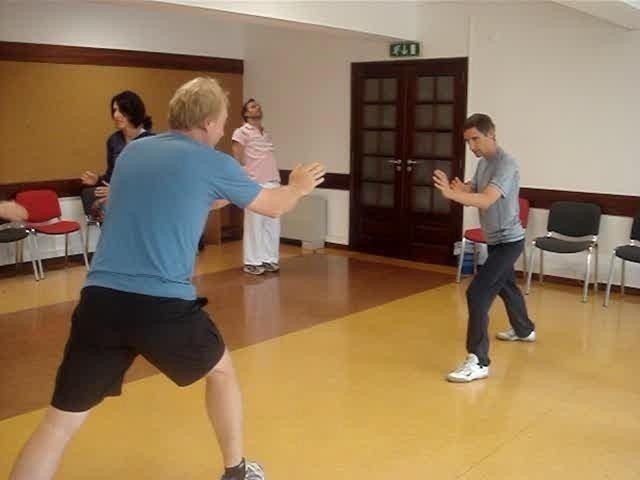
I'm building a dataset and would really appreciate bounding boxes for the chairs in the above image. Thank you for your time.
[80,184,101,265]
[601,210,640,310]
[16,190,91,281]
[1,203,40,282]
[456,193,532,288]
[523,202,601,302]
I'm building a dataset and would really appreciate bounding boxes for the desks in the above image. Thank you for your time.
[202,198,243,248]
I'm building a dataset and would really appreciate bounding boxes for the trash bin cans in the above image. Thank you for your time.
[454,242,482,274]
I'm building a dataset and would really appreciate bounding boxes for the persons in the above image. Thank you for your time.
[235,98,282,275]
[431,113,535,382]
[0,195,28,223]
[81,90,156,197]
[6,77,326,480]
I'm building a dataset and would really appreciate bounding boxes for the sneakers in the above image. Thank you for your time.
[495,327,536,342]
[242,265,265,274]
[220,457,265,480]
[446,353,489,383]
[263,262,280,272]
